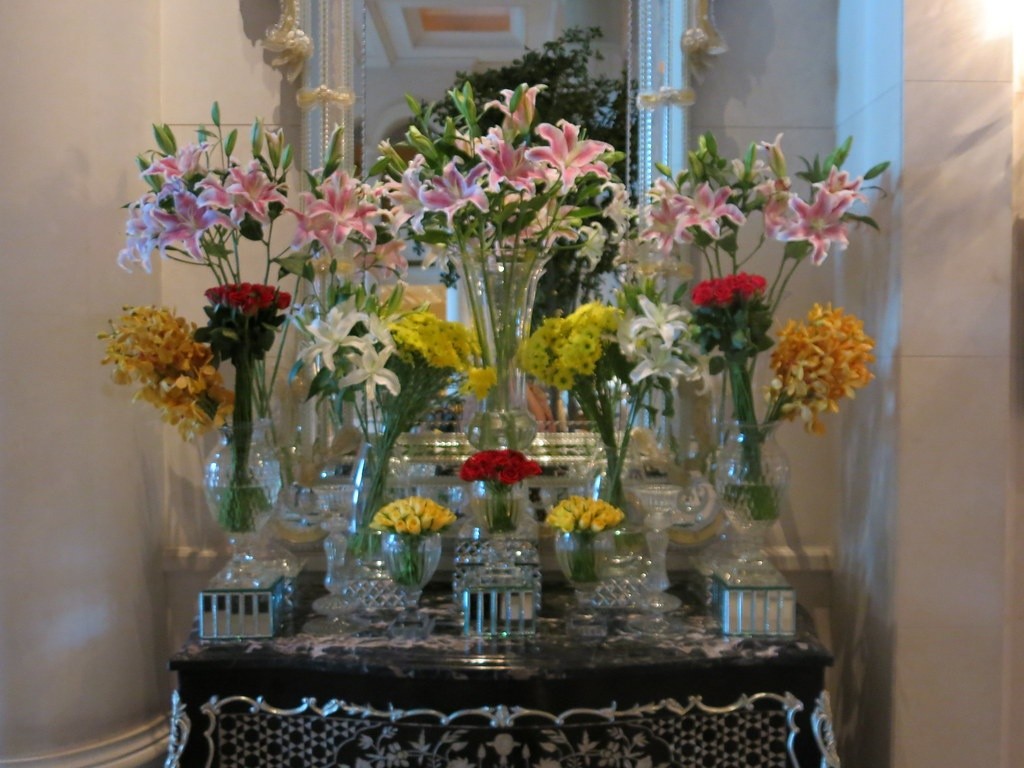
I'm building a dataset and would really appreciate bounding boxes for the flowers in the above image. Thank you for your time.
[95,78,892,589]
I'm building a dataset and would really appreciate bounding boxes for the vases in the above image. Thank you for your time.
[194,245,800,646]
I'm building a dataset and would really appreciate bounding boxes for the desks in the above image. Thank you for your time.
[159,632,848,768]
